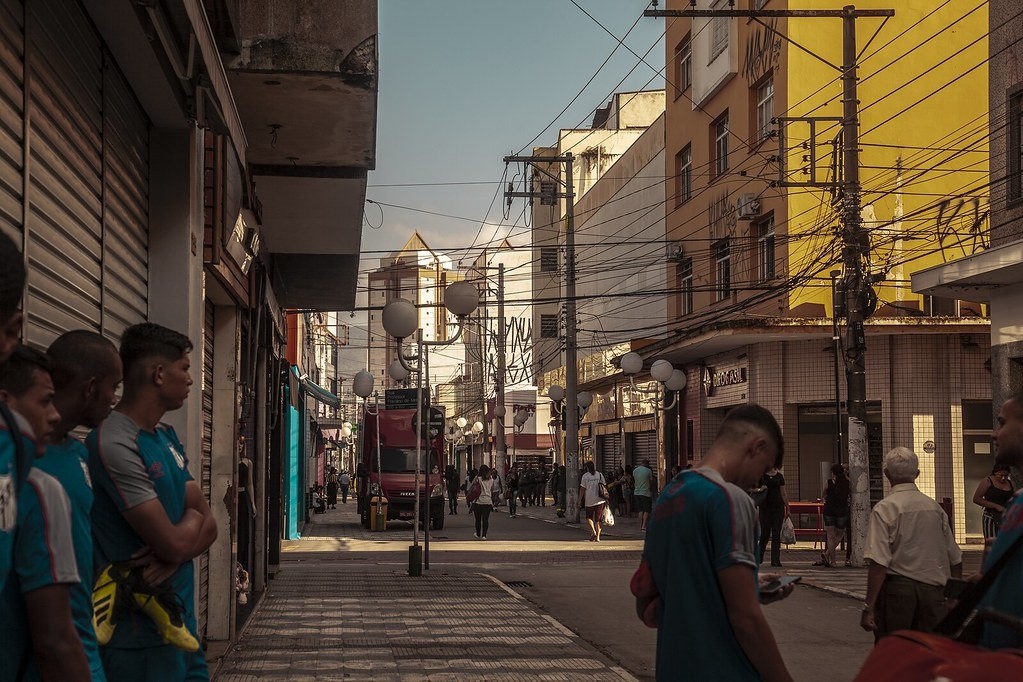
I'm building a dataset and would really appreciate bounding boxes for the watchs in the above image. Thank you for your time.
[758,488,761,493]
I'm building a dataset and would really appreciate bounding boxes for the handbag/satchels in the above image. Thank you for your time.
[749,477,768,505]
[779,516,796,545]
[598,472,610,500]
[629,555,662,628]
[461,484,467,491]
[505,489,513,499]
[601,505,615,527]
[338,488,343,494]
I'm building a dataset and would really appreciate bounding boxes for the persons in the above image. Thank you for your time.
[43,325,127,682]
[0,344,93,682]
[577,461,611,543]
[971,465,1017,547]
[0,226,38,598]
[446,458,693,517]
[860,446,965,651]
[630,401,798,682]
[972,390,1023,651]
[465,464,498,540]
[812,462,853,568]
[81,321,220,682]
[309,464,357,514]
[630,460,655,532]
[745,467,793,568]
[237,435,258,576]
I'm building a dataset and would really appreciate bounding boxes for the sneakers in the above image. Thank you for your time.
[90,561,131,645]
[122,580,200,653]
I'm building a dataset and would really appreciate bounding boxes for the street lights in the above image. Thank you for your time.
[352,370,386,532]
[494,404,529,465]
[621,352,686,499]
[381,283,479,576]
[443,418,484,469]
[549,385,594,431]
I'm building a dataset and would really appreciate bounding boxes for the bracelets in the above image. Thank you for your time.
[784,504,788,506]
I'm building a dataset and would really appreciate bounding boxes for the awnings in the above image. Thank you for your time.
[317,415,342,430]
[300,378,341,409]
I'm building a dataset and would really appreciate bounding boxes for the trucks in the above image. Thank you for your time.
[361,402,446,528]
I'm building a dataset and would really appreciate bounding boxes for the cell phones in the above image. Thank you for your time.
[944,577,970,600]
[758,576,802,596]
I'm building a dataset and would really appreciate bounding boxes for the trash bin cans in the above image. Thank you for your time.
[369,496,389,532]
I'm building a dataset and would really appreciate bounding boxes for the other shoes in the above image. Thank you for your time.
[510,515,516,518]
[557,512,565,518]
[590,535,600,542]
[618,513,630,519]
[640,528,646,532]
[760,553,837,568]
[326,506,336,509]
[521,502,556,508]
[449,511,457,515]
[492,508,498,512]
[474,532,487,541]
